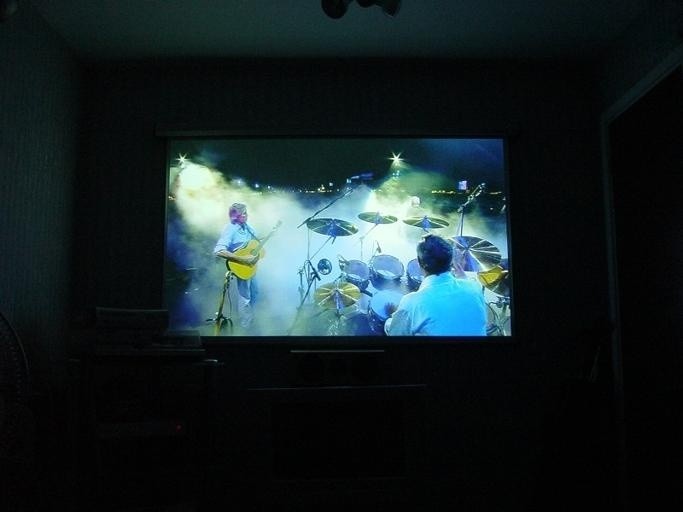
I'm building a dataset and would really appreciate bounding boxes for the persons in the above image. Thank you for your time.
[384,234,487,336]
[214,202,278,330]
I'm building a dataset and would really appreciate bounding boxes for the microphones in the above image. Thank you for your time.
[351,173,373,179]
[309,261,321,281]
[377,241,382,253]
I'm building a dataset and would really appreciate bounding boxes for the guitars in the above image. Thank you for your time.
[206,271,233,337]
[226,221,280,280]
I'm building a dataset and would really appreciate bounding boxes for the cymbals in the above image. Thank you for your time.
[403,215,449,229]
[316,283,360,310]
[358,212,397,224]
[477,258,511,298]
[308,218,358,236]
[446,236,501,273]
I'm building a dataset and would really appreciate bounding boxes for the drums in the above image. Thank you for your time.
[367,289,407,335]
[305,305,368,336]
[407,259,425,291]
[368,255,404,290]
[340,259,369,290]
